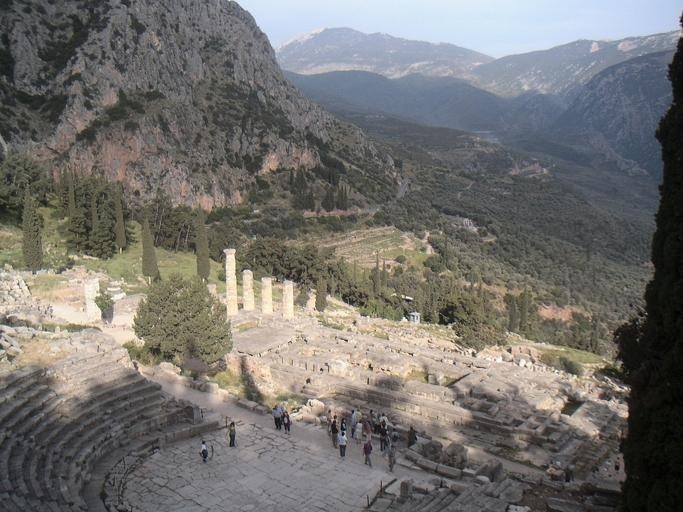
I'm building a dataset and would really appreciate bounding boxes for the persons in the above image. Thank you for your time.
[564,464,574,482]
[614,457,620,471]
[327,409,417,473]
[199,439,209,462]
[226,422,236,447]
[270,404,292,435]
